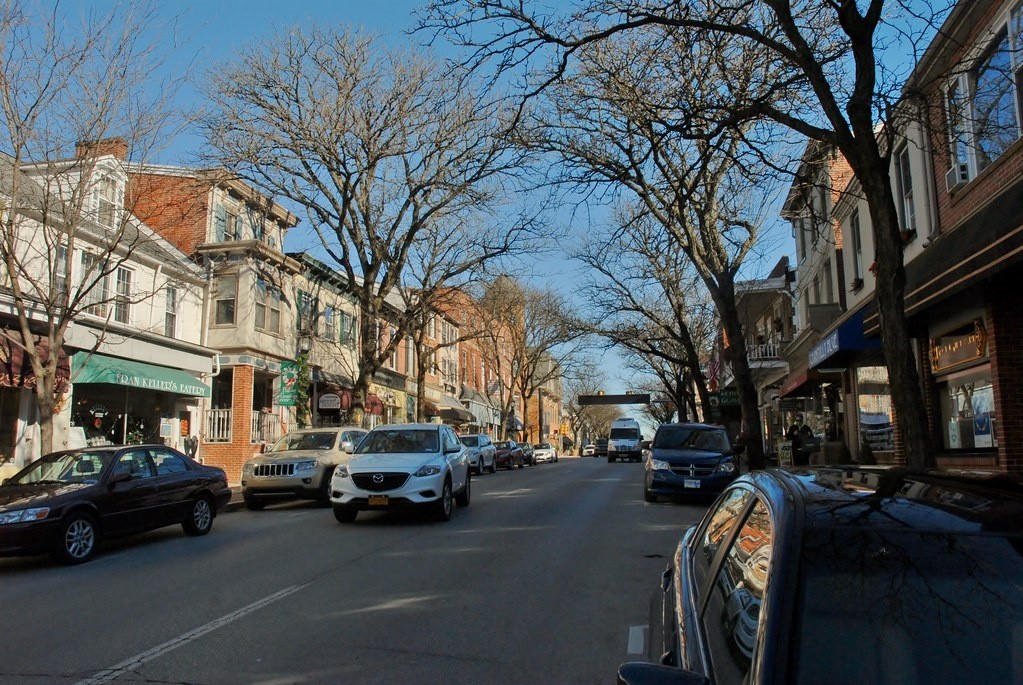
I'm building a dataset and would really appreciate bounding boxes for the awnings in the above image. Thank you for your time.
[311,384,384,416]
[0,326,70,393]
[501,413,524,431]
[440,395,476,422]
[72,351,210,398]
[809,311,887,370]
[863,183,1023,335]
[426,401,440,416]
[779,366,816,399]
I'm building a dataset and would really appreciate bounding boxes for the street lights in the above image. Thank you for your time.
[296,328,314,429]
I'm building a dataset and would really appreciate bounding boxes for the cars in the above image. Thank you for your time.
[492,441,524,470]
[594,439,608,458]
[517,442,537,466]
[531,443,559,464]
[240,428,386,511]
[0,443,232,566]
[643,423,743,503]
[581,445,595,456]
[329,423,472,523]
[618,463,1023,685]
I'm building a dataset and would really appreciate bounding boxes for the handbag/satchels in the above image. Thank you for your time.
[802,433,821,452]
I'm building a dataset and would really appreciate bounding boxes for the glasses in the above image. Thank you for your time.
[795,420,802,422]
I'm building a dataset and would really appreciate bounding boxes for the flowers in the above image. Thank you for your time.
[869,256,878,276]
[900,227,915,244]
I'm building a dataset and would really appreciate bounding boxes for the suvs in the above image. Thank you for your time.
[458,434,498,477]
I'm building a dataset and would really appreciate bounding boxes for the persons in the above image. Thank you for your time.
[402,432,437,450]
[554,430,573,456]
[787,414,814,466]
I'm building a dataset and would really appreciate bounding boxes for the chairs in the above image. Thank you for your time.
[68,459,99,483]
[158,458,181,473]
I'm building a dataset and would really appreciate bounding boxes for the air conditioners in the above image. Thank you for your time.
[945,163,967,194]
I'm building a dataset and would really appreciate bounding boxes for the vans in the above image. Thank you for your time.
[607,417,644,464]
